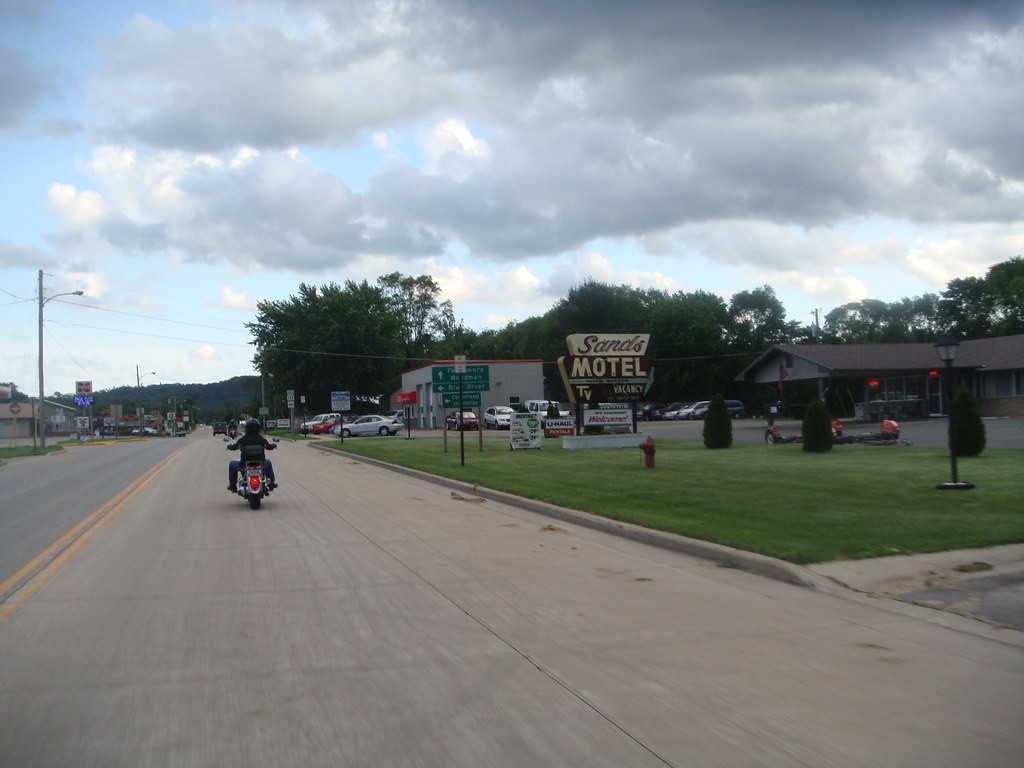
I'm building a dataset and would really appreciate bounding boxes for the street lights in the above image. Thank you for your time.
[137,372,155,436]
[935,333,959,483]
[39,291,84,448]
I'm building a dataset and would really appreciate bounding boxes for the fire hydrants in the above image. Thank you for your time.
[639,435,656,467]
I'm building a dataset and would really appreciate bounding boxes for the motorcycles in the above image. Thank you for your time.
[226,425,238,439]
[223,437,281,510]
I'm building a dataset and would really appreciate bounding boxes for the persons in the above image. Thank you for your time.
[228,420,238,432]
[226,417,278,498]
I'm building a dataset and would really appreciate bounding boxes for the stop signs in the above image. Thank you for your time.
[9,403,21,414]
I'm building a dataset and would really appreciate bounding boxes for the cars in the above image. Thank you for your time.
[213,422,227,436]
[636,401,710,421]
[311,416,354,435]
[484,405,516,430]
[446,411,480,432]
[386,409,404,423]
[335,415,405,438]
[688,399,745,420]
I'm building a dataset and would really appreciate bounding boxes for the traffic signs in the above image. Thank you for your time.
[432,365,490,383]
[432,382,490,393]
[441,392,482,408]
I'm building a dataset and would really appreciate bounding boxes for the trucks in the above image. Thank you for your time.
[524,400,570,429]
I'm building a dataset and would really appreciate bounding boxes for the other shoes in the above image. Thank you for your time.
[268,483,278,491]
[227,486,237,490]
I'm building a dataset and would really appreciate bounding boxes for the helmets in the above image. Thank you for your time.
[231,419,234,424]
[245,418,261,434]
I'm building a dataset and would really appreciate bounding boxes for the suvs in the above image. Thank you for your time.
[300,413,341,434]
